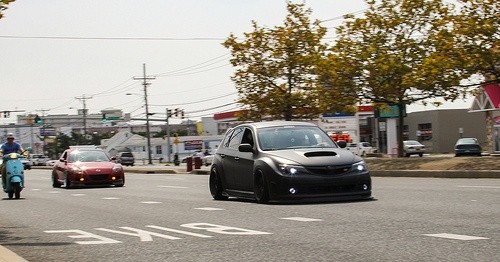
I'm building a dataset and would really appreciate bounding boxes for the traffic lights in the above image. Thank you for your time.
[34,115,41,123]
[167,109,171,117]
[181,111,184,119]
[175,108,178,116]
[102,113,106,122]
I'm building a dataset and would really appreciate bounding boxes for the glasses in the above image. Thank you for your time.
[7,137,15,140]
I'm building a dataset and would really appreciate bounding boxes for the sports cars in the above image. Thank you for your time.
[208,120,372,204]
[51,147,125,189]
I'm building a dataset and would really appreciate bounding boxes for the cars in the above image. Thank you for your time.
[454,137,482,157]
[0,153,60,170]
[359,141,378,158]
[203,149,217,167]
[182,152,205,163]
[402,140,426,157]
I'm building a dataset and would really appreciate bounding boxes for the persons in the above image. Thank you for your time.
[416,126,422,143]
[0,133,27,189]
[205,150,211,166]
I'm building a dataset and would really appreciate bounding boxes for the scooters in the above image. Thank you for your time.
[0,147,32,199]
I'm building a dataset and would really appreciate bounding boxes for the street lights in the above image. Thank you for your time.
[126,92,152,164]
[69,106,87,145]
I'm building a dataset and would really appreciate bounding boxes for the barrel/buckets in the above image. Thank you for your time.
[186,157,192,172]
[194,157,200,169]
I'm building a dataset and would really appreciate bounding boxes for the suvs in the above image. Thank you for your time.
[116,152,135,166]
